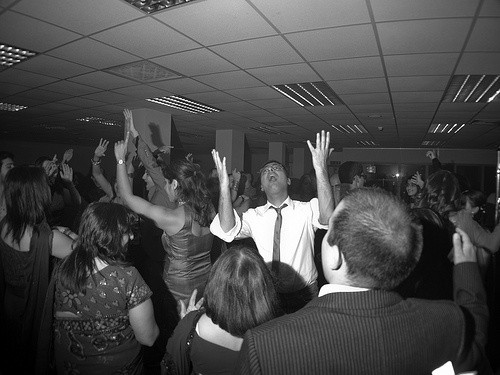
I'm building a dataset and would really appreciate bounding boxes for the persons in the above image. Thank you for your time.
[0,163,85,374]
[3,107,498,323]
[233,186,489,373]
[154,246,285,375]
[36,202,161,374]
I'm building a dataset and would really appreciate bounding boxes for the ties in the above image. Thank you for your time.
[270,204,288,275]
